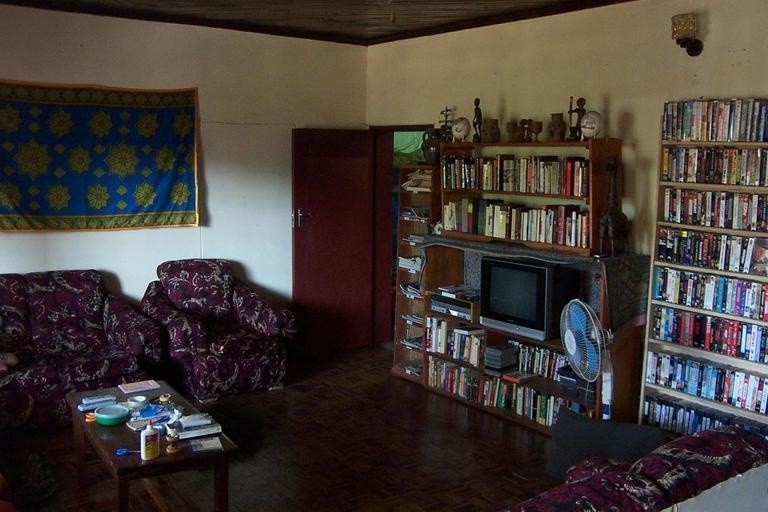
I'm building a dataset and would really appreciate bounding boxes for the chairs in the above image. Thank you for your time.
[141,258,306,407]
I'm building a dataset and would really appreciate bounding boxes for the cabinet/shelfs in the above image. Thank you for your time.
[421,299,645,444]
[390,162,465,400]
[635,98,768,446]
[434,136,624,257]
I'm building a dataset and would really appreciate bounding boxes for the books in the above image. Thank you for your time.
[643,96,768,436]
[399,170,431,375]
[118,379,160,394]
[443,157,588,248]
[164,414,222,439]
[424,286,571,426]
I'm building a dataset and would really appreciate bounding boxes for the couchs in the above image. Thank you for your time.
[500,424,768,511]
[0,268,166,432]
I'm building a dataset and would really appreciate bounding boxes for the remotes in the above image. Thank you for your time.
[77,400,116,411]
[82,394,116,405]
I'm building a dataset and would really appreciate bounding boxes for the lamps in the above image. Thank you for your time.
[671,11,706,58]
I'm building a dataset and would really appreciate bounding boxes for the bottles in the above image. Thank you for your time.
[140,418,160,461]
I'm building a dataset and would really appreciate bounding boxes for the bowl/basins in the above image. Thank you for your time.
[95,406,129,425]
[126,395,148,409]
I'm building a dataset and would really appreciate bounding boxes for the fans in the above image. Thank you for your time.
[558,297,616,419]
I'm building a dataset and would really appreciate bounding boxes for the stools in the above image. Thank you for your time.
[548,414,665,482]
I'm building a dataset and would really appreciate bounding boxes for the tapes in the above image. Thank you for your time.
[127,396,147,407]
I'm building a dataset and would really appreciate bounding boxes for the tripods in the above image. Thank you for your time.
[480,256,586,342]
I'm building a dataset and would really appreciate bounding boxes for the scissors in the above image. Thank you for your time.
[112,448,141,456]
[85,413,96,423]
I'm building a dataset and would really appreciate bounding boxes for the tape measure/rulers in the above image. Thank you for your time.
[158,393,172,402]
